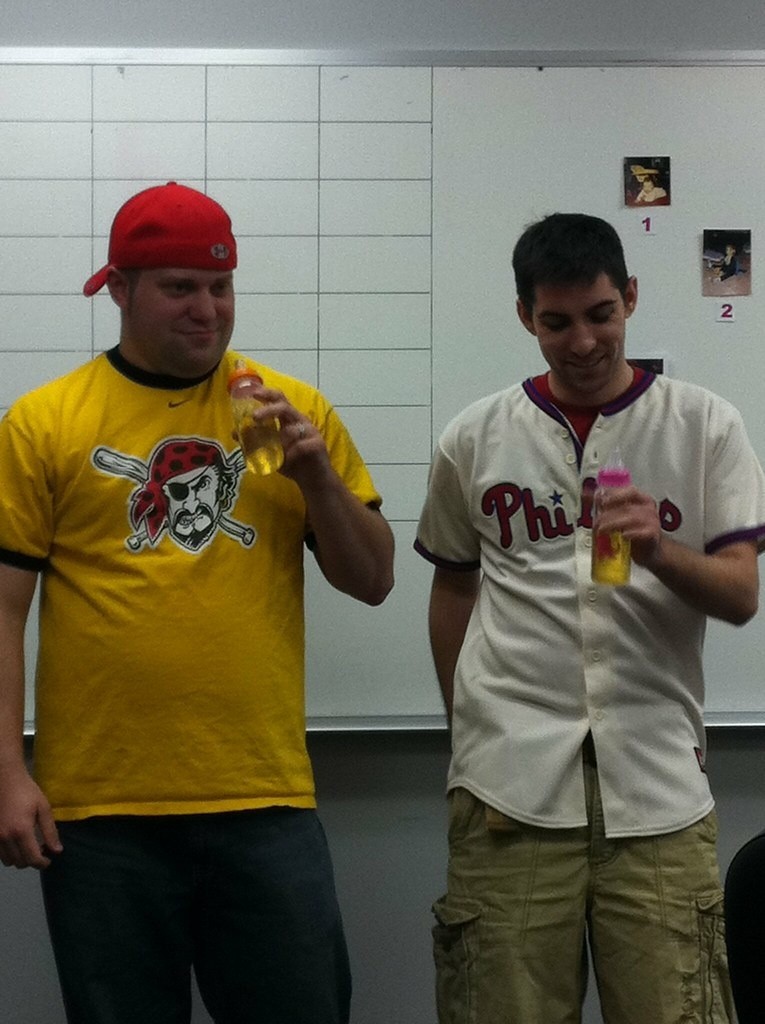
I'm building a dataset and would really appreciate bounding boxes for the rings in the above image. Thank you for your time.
[294,420,306,439]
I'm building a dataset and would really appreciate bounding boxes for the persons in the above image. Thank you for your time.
[1,182,395,1023]
[413,214,765,1024]
[708,246,739,282]
[635,177,667,203]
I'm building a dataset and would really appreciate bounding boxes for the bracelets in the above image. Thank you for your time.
[630,532,661,566]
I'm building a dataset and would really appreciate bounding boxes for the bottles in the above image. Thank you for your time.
[226,358,285,475]
[591,448,633,585]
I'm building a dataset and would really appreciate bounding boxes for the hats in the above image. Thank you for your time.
[83,181,238,299]
